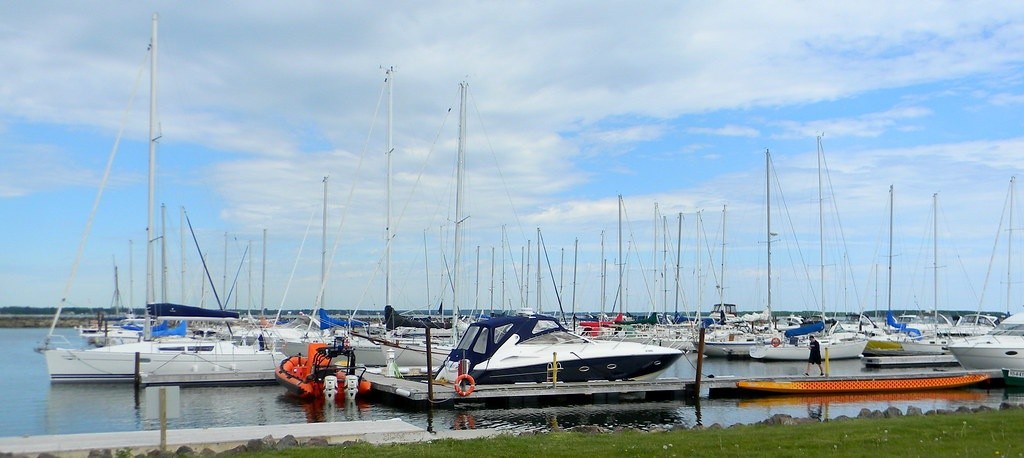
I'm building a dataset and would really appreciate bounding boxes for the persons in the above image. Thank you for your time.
[804,336,825,376]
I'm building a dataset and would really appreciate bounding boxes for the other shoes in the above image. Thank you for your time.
[819,373,824,376]
[802,373,810,377]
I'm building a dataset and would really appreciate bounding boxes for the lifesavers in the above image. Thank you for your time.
[454,373,475,397]
[771,337,781,347]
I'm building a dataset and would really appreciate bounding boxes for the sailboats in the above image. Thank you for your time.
[31,12,1024,412]
